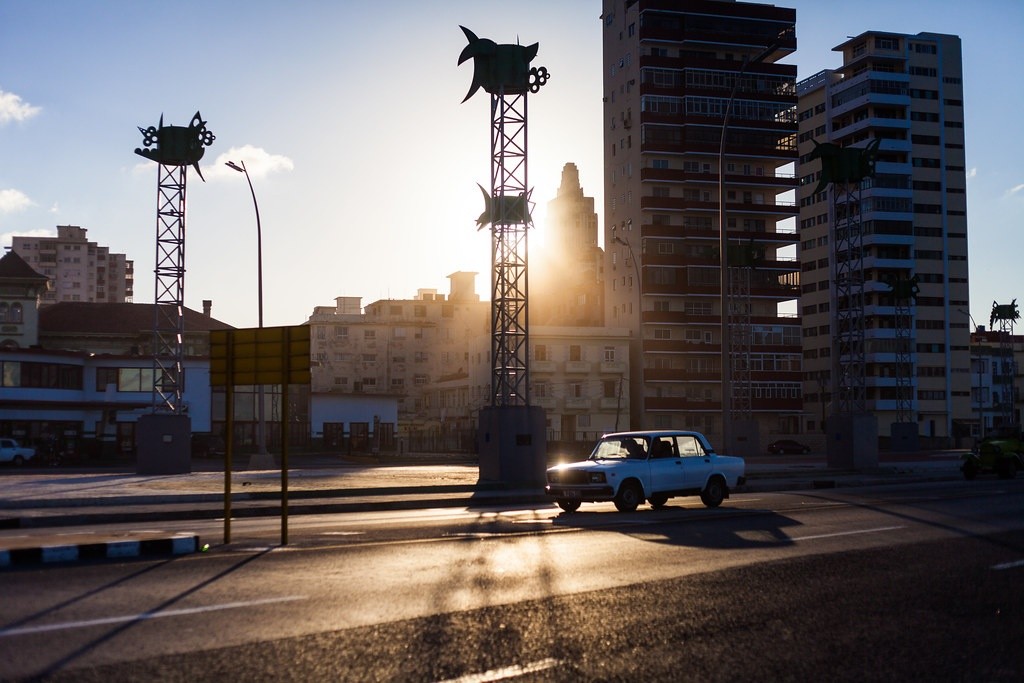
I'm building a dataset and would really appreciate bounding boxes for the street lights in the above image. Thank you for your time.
[225,158,269,455]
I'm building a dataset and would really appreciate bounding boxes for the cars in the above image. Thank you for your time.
[545,431,747,515]
[0,439,36,466]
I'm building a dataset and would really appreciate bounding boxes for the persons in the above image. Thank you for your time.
[626,437,669,458]
